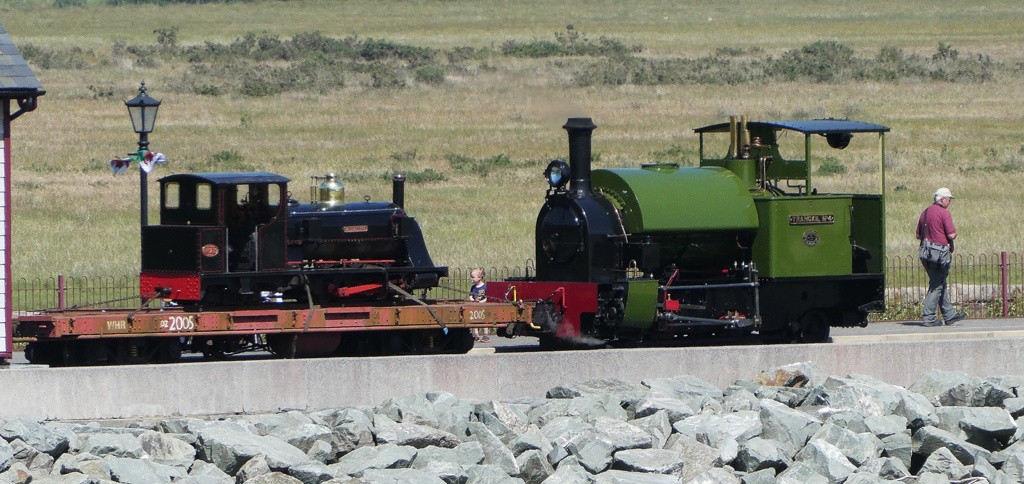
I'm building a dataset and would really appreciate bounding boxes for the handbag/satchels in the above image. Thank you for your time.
[919,239,951,267]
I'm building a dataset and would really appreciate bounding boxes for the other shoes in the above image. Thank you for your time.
[922,319,943,326]
[945,312,966,325]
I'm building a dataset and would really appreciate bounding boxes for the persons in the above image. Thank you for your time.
[916,187,967,327]
[469,267,491,343]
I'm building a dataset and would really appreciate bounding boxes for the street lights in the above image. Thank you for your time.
[120,78,164,307]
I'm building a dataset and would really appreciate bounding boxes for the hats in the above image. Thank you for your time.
[934,187,955,202]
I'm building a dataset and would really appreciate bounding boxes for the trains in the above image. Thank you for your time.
[11,115,892,367]
